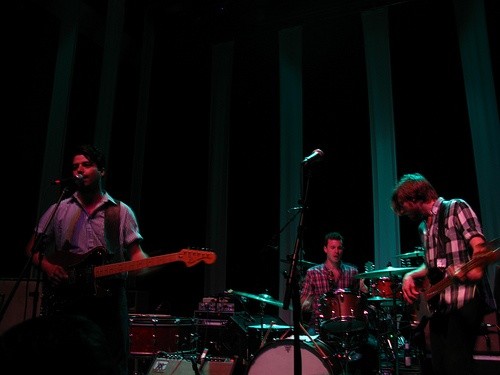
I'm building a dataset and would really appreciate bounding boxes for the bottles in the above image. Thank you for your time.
[403,342,411,368]
[379,349,390,375]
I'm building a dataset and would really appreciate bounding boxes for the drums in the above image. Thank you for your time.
[247,324,294,361]
[320,288,369,332]
[376,276,405,309]
[246,334,343,375]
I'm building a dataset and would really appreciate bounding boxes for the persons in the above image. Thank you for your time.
[391,173,486,375]
[300,232,368,312]
[26,143,150,375]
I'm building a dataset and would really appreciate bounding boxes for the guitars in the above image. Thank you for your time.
[409,239,500,329]
[47,246,217,313]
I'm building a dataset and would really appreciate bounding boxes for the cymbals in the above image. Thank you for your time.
[354,267,418,279]
[280,259,318,266]
[231,291,293,311]
[392,250,425,258]
[367,296,398,301]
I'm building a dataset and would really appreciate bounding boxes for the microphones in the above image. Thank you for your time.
[55,174,85,186]
[300,148,324,167]
[479,322,495,329]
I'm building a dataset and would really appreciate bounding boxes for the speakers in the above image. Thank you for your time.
[146,355,244,375]
[472,309,500,357]
